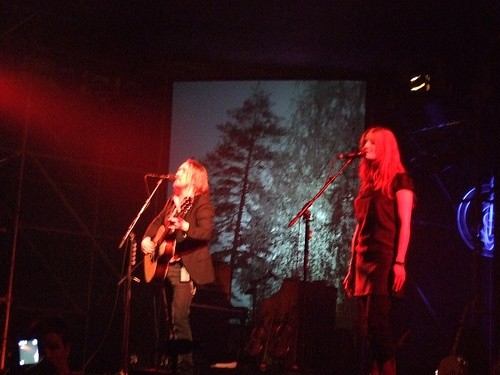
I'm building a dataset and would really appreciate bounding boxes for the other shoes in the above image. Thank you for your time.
[178,361,193,375]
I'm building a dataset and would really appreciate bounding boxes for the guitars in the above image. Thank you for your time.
[144,194,194,285]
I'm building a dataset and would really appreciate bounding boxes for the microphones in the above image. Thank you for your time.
[337,150,366,159]
[147,173,177,182]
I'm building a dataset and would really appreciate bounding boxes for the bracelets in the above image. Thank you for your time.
[394,261,405,266]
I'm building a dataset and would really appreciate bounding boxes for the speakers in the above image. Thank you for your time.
[186,303,247,370]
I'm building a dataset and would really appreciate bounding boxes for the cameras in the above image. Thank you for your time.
[18,338,40,367]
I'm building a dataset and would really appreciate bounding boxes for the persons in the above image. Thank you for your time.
[140,159,215,375]
[29,328,84,375]
[343,129,415,375]
